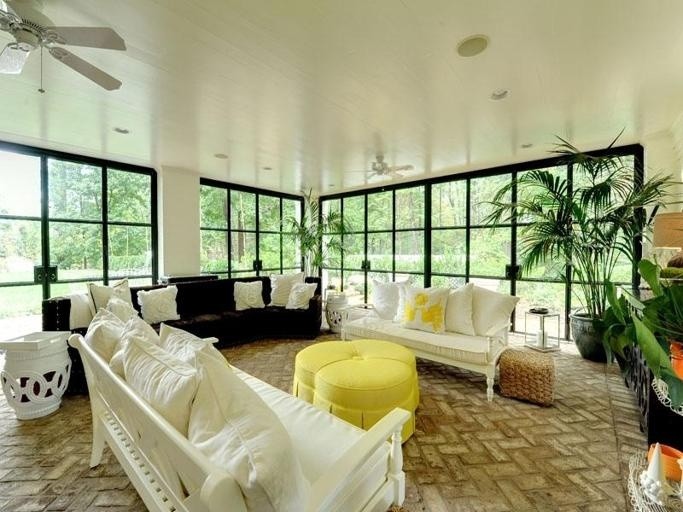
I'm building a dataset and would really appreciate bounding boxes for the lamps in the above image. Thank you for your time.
[475,124,683,363]
[652,212,683,250]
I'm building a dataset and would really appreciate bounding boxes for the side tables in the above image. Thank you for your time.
[326,294,349,333]
[0,331,73,420]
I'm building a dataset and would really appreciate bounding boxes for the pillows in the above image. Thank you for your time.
[88,276,230,439]
[189,350,301,511]
[370,279,519,347]
[286,283,317,310]
[234,280,266,311]
[267,273,306,307]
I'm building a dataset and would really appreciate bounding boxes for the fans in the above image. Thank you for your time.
[0,1,125,93]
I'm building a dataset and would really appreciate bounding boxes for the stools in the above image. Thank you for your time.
[500,350,555,407]
[292,339,421,445]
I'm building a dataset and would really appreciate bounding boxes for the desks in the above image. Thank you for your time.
[623,333,683,452]
[524,311,560,353]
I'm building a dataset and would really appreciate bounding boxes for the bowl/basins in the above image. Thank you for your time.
[646,443,682,480]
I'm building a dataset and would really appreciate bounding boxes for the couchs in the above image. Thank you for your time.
[338,303,513,403]
[42,276,323,395]
[68,333,412,512]
